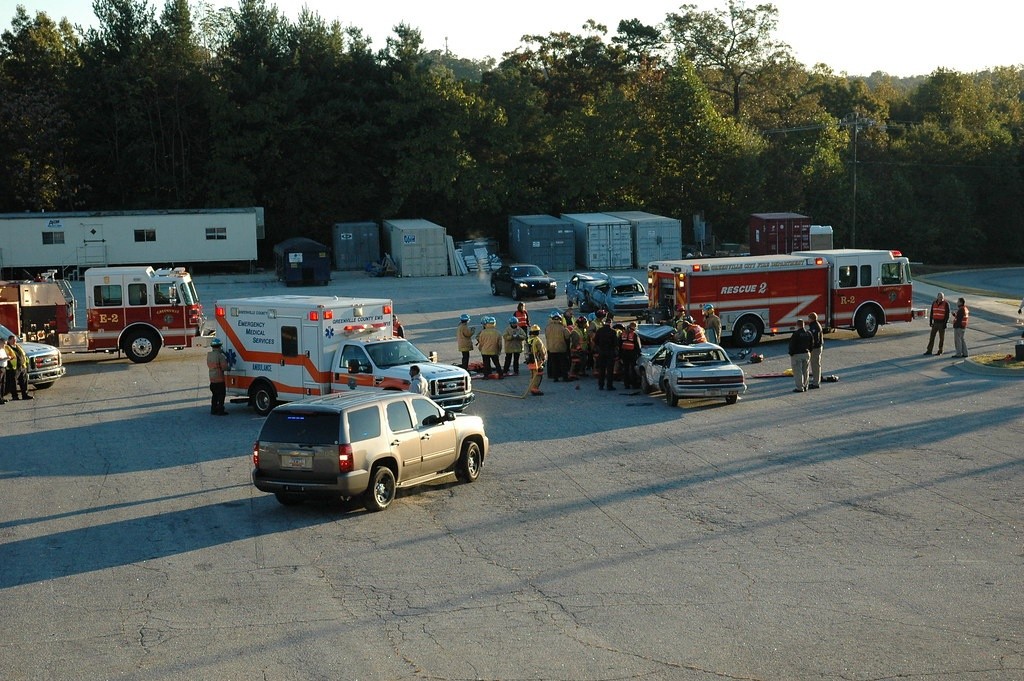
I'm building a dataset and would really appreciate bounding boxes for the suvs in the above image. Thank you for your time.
[252,392,489,512]
[0,325,67,386]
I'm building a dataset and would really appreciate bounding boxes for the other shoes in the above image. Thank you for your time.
[951,355,958,358]
[599,386,604,389]
[809,384,819,389]
[935,351,942,355]
[482,370,520,379]
[11,395,19,400]
[607,386,617,391]
[0,398,9,405]
[962,355,968,357]
[793,388,804,392]
[22,393,35,400]
[923,351,932,355]
[530,386,544,395]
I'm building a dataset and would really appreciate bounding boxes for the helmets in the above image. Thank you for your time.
[702,304,714,311]
[210,338,223,346]
[548,308,564,319]
[508,316,518,324]
[576,317,587,328]
[595,310,607,318]
[459,313,470,320]
[485,317,496,326]
[480,316,489,324]
[529,324,541,332]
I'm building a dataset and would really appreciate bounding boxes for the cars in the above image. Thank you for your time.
[635,340,748,405]
[565,272,650,319]
[489,264,557,299]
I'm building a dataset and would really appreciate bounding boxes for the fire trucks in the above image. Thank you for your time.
[649,248,928,349]
[1,265,217,365]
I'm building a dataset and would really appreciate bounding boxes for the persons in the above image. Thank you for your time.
[1018,298,1024,314]
[503,317,527,374]
[4,335,35,399]
[457,313,475,371]
[524,324,546,395]
[949,297,969,358]
[206,338,231,416]
[392,314,405,338]
[0,338,12,405]
[547,305,643,392]
[512,301,530,342]
[788,312,824,393]
[923,292,951,355]
[669,304,722,346]
[407,365,429,396]
[475,315,505,381]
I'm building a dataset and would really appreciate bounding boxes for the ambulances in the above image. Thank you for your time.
[213,294,476,416]
